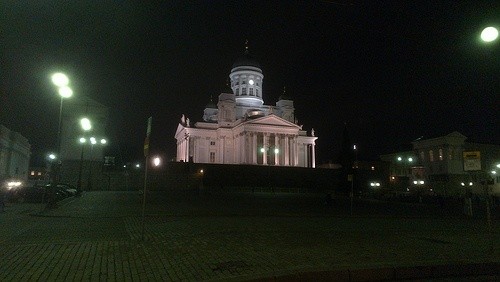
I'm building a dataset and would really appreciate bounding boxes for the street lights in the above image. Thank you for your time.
[76,119,91,195]
[46,72,74,208]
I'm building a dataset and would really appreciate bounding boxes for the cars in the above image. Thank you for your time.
[0,180,77,208]
